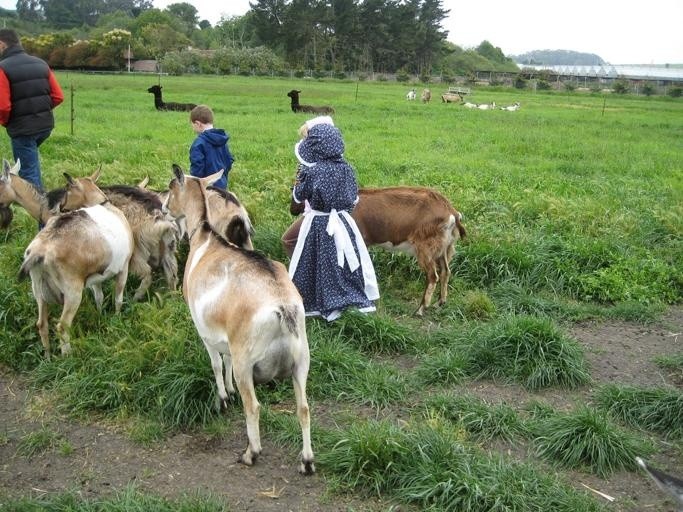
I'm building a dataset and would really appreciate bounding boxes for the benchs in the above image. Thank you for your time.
[458,87,470,96]
[447,87,459,94]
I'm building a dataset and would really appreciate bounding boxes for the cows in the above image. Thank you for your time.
[441,92,463,104]
[420,88,432,105]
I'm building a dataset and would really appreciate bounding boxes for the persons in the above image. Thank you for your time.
[188,104,232,196]
[291,117,378,322]
[0,29,63,197]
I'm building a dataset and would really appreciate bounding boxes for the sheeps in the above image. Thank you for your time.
[348,185,468,320]
[0,158,256,360]
[478,101,496,111]
[162,163,316,475]
[499,101,520,112]
[459,102,479,110]
[406,88,416,100]
